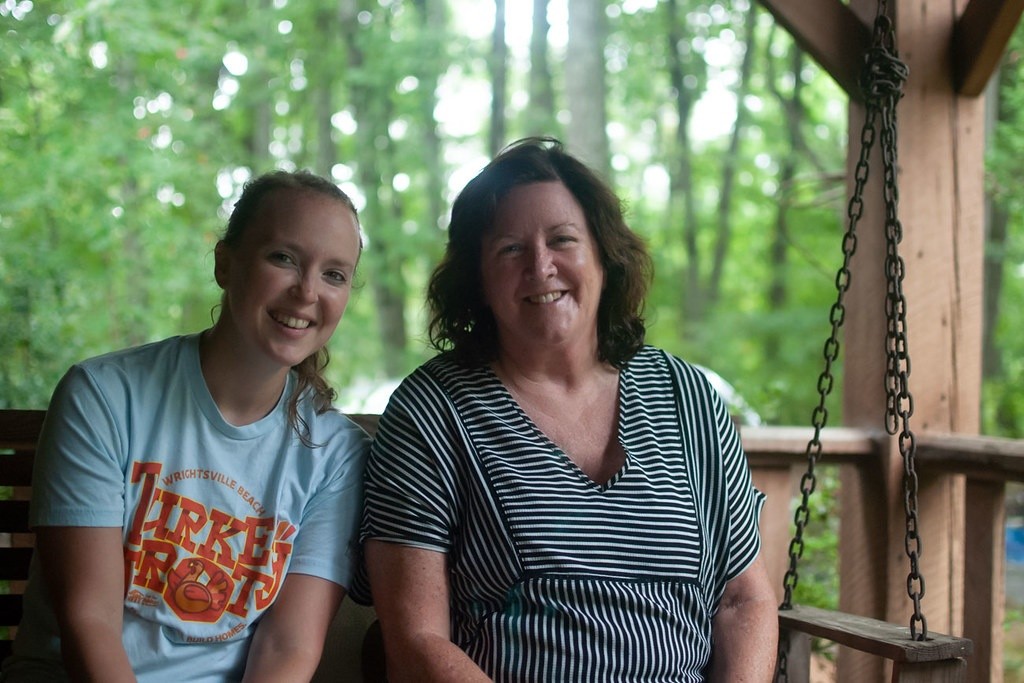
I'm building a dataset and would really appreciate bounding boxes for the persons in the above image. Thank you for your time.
[358,138,781,683]
[39,169,368,683]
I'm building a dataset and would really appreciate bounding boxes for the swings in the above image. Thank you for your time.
[0,0,974,683]
